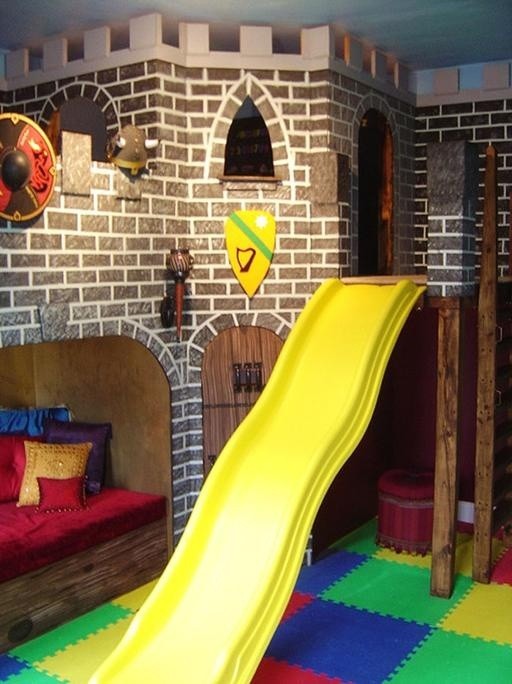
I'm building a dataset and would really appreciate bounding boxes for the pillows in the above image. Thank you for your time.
[1,404,114,514]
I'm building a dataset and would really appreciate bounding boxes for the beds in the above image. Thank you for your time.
[1,487,167,652]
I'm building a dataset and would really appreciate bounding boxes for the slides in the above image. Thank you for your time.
[86,276,429,683]
